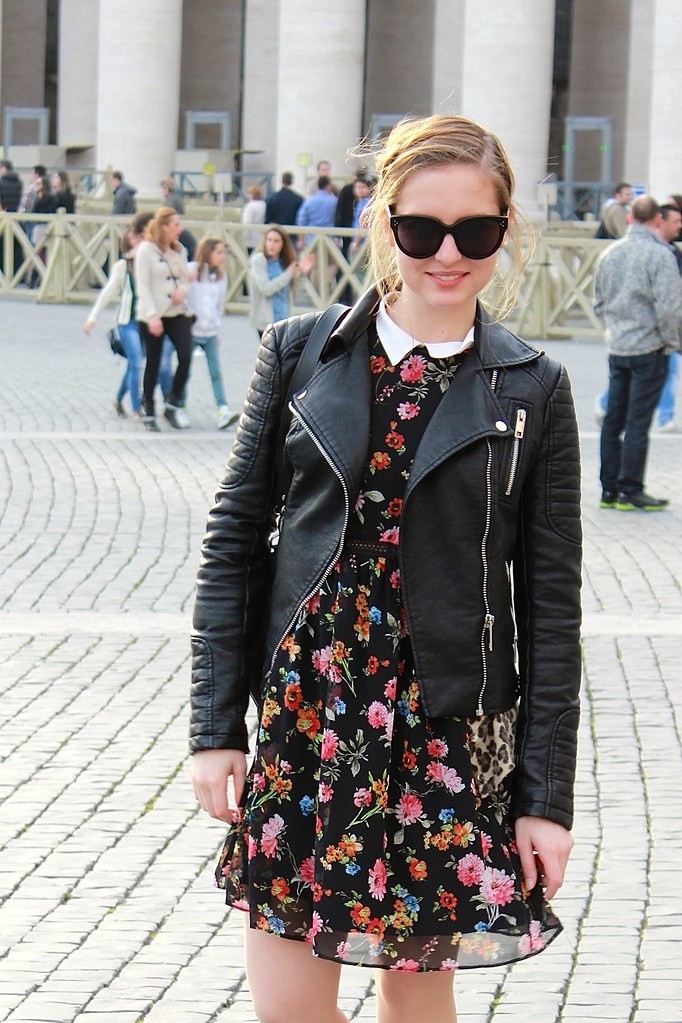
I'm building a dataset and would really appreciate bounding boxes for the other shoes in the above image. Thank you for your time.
[614,491,668,512]
[599,490,619,508]
[107,399,126,418]
[164,407,184,429]
[216,411,241,429]
[142,417,159,431]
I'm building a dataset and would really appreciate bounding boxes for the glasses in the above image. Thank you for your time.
[388,203,510,260]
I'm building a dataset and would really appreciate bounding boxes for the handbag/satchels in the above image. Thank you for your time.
[106,328,126,358]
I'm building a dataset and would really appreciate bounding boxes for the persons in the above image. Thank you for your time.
[88,170,138,288]
[592,194,682,512]
[162,180,185,215]
[239,159,378,307]
[189,116,583,1023]
[83,207,241,434]
[668,195,682,241]
[0,159,78,289]
[249,228,317,340]
[600,182,634,238]
[594,204,682,433]
